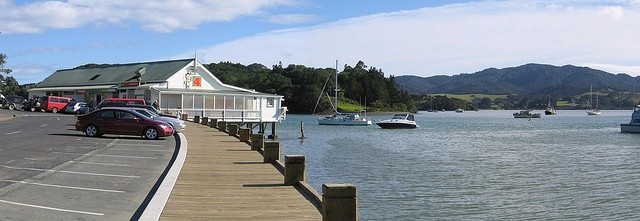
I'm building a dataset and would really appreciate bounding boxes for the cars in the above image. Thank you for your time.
[126,104,177,118]
[133,108,186,134]
[72,102,94,115]
[75,108,174,140]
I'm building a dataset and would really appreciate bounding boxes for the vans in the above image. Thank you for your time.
[95,98,146,109]
[40,96,76,114]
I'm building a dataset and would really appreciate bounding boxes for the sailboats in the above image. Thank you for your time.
[312,60,372,126]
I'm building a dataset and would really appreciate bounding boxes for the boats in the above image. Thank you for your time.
[545,111,556,115]
[455,109,464,113]
[587,111,601,115]
[621,105,640,133]
[376,112,417,128]
[514,110,541,118]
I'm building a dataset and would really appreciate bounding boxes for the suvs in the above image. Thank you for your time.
[2,96,25,110]
